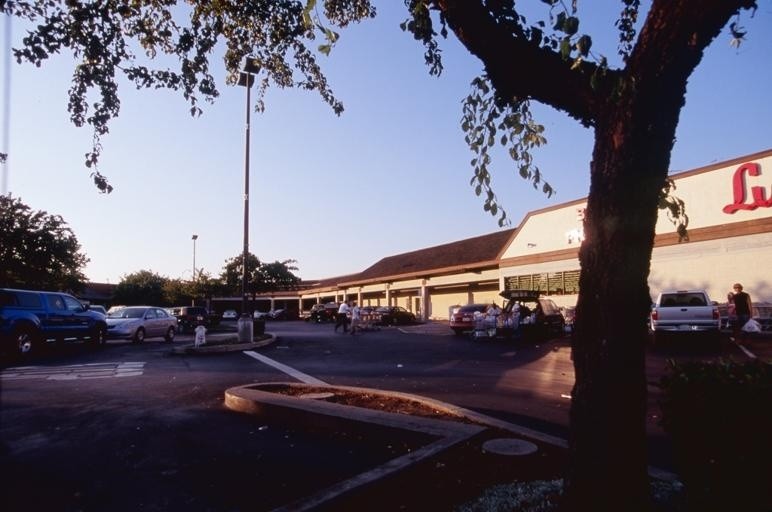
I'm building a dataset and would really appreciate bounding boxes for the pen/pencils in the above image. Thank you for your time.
[0,286,109,362]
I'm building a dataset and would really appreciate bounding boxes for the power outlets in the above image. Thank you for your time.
[649,289,772,344]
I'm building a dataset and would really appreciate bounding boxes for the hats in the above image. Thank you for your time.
[310,301,383,326]
[369,306,417,326]
[448,302,503,334]
[105,305,179,345]
[165,305,301,334]
[499,288,577,340]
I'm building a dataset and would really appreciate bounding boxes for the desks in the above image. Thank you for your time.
[237,55,263,318]
[192,234,198,285]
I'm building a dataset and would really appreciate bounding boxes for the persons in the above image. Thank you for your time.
[350,301,360,336]
[471,300,521,339]
[726,291,736,319]
[726,282,753,346]
[334,299,352,333]
